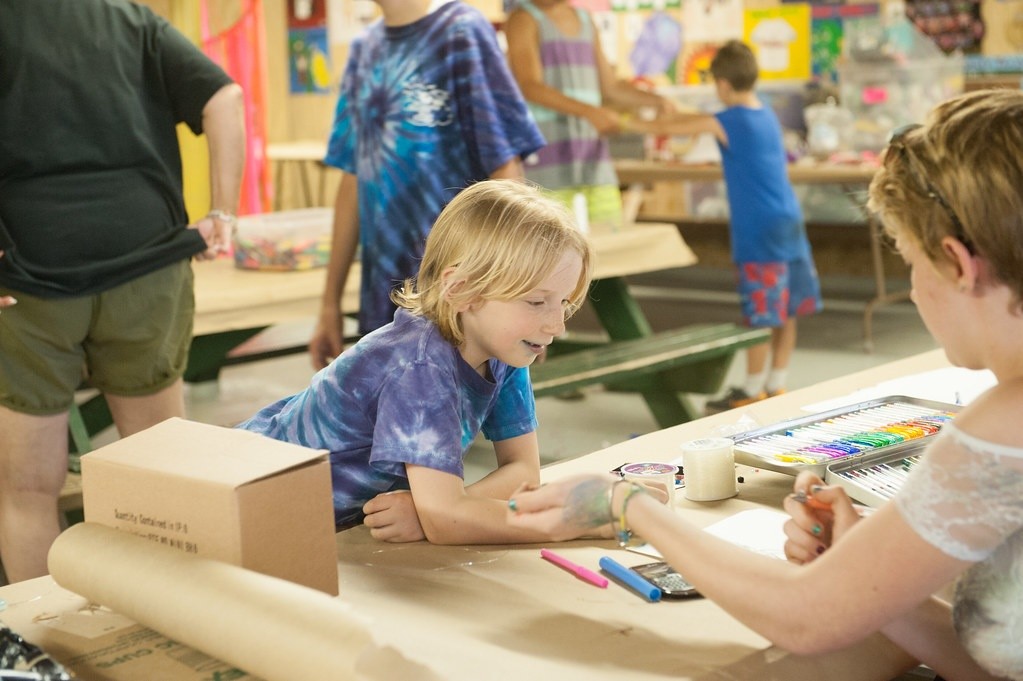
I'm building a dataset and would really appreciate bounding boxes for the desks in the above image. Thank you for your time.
[614,159,908,355]
[72,208,698,446]
[1,349,1022,681]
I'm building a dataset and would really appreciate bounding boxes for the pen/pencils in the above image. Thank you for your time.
[734,402,962,501]
[540,545,609,588]
[791,493,878,516]
[599,557,661,602]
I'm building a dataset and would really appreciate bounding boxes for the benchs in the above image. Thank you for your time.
[527,308,773,429]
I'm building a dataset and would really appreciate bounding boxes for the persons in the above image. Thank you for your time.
[0,0,247,587]
[612,39,824,415]
[309,0,547,376]
[232,176,668,546]
[505,1,676,228]
[506,83,1023,681]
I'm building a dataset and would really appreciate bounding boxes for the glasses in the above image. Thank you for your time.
[882,123,977,256]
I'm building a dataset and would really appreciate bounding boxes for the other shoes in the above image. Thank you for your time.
[706,389,766,419]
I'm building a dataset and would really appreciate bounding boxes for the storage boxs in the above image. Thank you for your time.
[81,416,339,597]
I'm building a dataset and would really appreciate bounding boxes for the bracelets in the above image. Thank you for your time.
[207,209,237,223]
[617,109,635,135]
[609,476,643,548]
